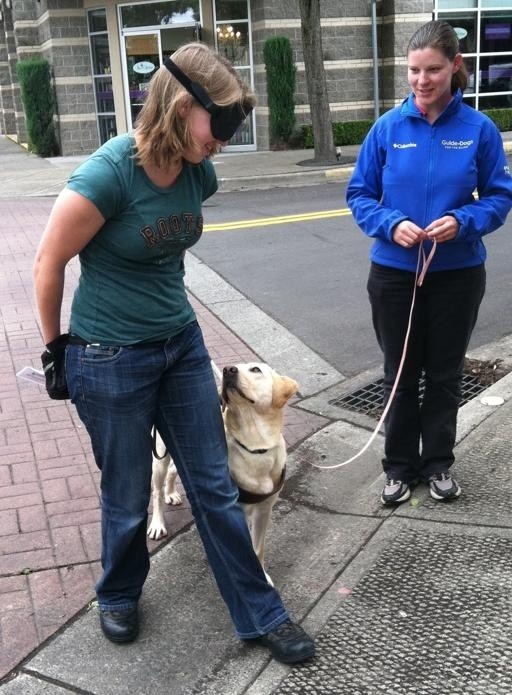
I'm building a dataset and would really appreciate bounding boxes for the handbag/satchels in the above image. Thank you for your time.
[41,333,70,400]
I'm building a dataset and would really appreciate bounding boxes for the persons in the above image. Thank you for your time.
[31,40,315,665]
[345,19,512,506]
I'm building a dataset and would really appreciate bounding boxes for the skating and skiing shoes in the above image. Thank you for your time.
[421,472,462,501]
[381,475,423,505]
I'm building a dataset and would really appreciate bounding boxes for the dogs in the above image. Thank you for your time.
[146,359,299,589]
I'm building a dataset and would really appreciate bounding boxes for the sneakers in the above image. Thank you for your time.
[241,619,315,662]
[99,610,139,644]
